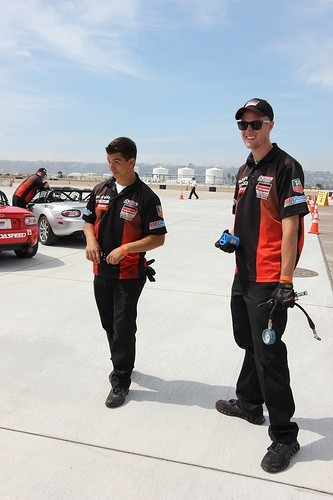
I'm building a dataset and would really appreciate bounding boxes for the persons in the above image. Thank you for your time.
[187,177,200,199]
[215,97,308,474]
[13,168,50,208]
[83,138,167,407]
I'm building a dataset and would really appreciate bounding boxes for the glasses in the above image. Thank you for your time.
[237,120,271,131]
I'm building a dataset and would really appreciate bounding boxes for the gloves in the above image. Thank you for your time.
[144,258,156,282]
[214,229,235,253]
[268,282,295,309]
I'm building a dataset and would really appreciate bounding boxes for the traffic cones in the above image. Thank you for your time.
[179,189,184,200]
[305,195,321,235]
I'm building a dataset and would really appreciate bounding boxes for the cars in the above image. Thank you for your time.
[0,191,39,258]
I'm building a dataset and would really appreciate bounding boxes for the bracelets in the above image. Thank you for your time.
[280,274,291,281]
[120,245,127,256]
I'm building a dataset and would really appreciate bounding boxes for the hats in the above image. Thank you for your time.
[234,98,274,122]
[38,168,47,177]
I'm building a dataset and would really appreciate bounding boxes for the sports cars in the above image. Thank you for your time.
[27,185,93,246]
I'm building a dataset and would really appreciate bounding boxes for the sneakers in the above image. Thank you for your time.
[215,398,266,425]
[260,439,300,474]
[108,369,115,383]
[105,385,130,409]
